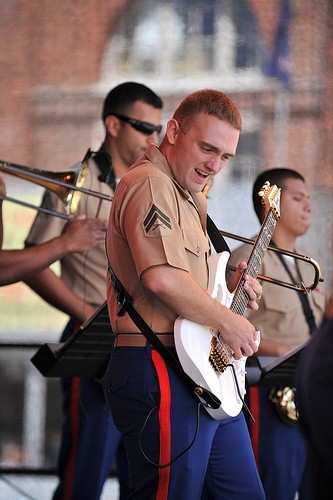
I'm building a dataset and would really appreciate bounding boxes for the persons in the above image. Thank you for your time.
[0,171,108,324]
[225,168,325,499]
[104,88,268,499]
[21,82,163,500]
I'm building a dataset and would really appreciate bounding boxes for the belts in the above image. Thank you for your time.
[113,333,174,348]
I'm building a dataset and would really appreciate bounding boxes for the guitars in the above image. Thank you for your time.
[162,180,285,422]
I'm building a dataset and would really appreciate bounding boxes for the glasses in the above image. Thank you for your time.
[109,111,162,136]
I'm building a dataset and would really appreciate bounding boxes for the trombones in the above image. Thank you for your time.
[0,157,326,297]
